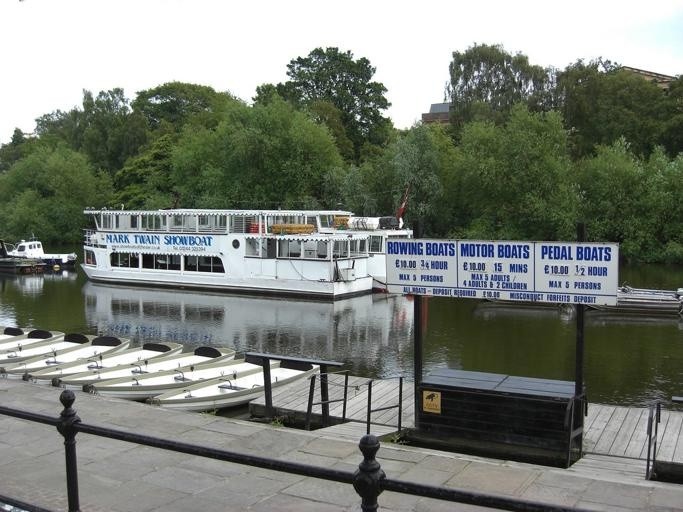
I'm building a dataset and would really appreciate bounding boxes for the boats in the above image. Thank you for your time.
[2,335,131,381]
[79,206,418,303]
[83,353,283,401]
[78,280,413,357]
[0,233,78,276]
[0,326,37,343]
[1,329,66,353]
[485,275,682,316]
[21,341,184,384]
[0,335,100,367]
[146,359,321,410]
[51,346,236,389]
